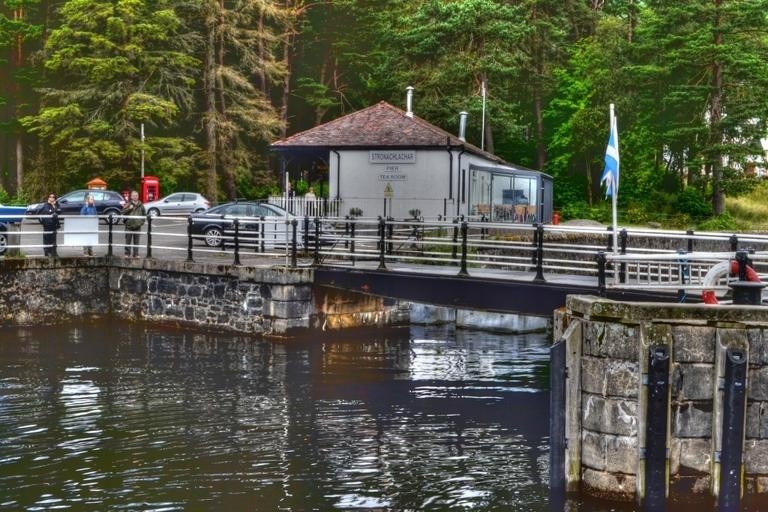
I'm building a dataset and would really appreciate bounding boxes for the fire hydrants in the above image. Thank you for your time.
[552,212,559,225]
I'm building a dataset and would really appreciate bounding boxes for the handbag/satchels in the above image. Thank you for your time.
[122,218,127,223]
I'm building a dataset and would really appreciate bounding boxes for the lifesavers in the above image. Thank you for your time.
[702,260,760,304]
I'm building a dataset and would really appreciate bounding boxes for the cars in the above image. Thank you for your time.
[0,198,27,259]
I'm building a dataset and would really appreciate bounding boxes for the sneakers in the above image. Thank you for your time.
[125,255,141,259]
[84,253,96,257]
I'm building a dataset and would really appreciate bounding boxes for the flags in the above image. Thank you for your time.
[600,115,619,200]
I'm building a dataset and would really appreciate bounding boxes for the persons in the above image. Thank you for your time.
[42,193,61,256]
[80,194,97,256]
[518,194,528,222]
[120,191,146,258]
[305,186,317,216]
